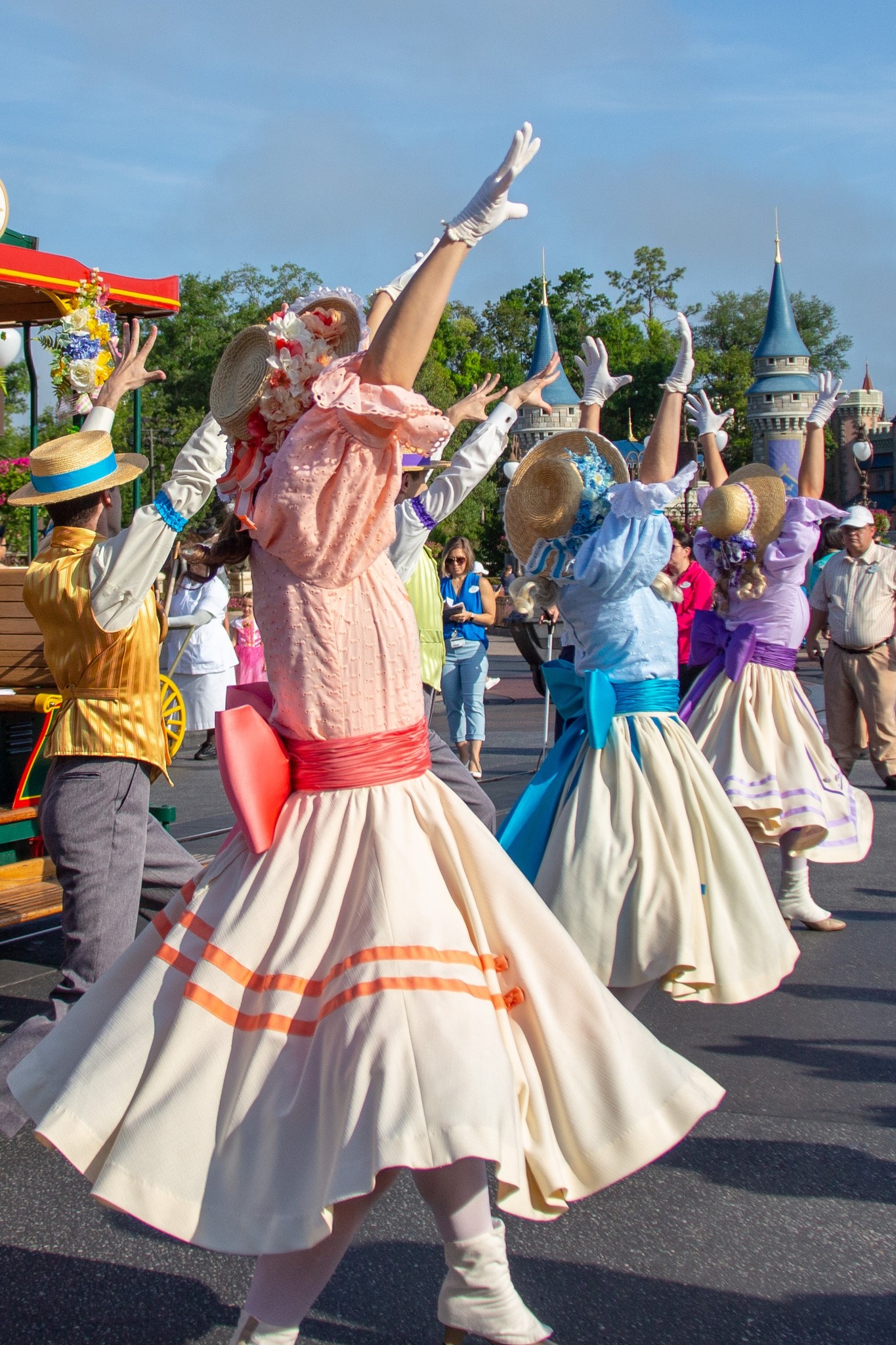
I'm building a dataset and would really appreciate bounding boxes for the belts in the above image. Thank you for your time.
[832,640,878,654]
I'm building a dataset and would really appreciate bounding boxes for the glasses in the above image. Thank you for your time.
[672,544,681,552]
[446,555,466,565]
[1,542,10,550]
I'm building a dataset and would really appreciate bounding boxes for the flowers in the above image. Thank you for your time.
[23,267,124,422]
[564,437,618,544]
[217,301,344,457]
[696,531,757,579]
[870,509,892,536]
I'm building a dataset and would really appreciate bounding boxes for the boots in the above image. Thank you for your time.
[778,864,844,929]
[227,1310,299,1345]
[439,1219,557,1345]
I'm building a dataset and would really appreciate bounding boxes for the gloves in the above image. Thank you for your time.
[684,389,734,438]
[804,371,850,427]
[453,120,541,249]
[369,238,443,302]
[657,310,696,393]
[573,335,634,407]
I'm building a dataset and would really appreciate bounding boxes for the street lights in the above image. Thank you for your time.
[503,434,523,578]
[852,419,874,508]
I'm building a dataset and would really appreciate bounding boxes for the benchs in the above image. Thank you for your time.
[0,567,63,810]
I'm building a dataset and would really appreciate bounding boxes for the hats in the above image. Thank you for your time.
[9,428,149,508]
[838,506,875,528]
[503,430,632,582]
[472,561,489,576]
[209,298,362,446]
[697,462,787,574]
[401,430,452,471]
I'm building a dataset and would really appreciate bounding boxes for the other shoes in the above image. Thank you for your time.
[885,775,896,788]
[194,739,217,760]
[463,759,470,772]
[485,677,500,690]
[469,760,482,778]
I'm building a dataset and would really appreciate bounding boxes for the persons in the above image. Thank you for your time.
[165,518,267,761]
[538,605,559,623]
[2,318,228,1140]
[807,504,896,790]
[685,370,874,928]
[494,308,802,1011]
[387,349,561,836]
[208,120,561,1345]
[664,530,716,705]
[473,561,500,690]
[439,537,496,777]
[500,564,516,595]
[809,522,847,649]
